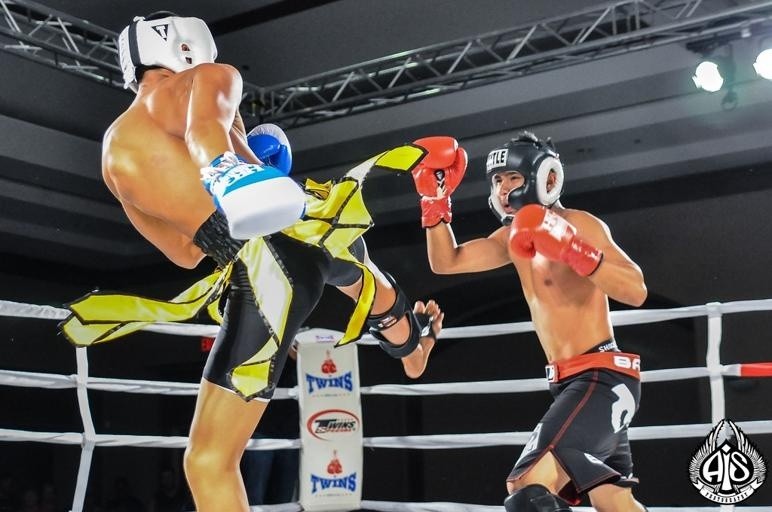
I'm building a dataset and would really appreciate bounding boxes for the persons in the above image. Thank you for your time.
[97,9,446,512]
[407,130,650,512]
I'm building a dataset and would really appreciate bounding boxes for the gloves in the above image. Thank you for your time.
[237,121,293,176]
[200,150,307,241]
[411,135,468,229]
[508,203,603,279]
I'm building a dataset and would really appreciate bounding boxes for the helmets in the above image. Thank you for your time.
[485,141,565,227]
[118,15,218,93]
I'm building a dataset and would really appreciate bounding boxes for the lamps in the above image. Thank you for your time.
[689,49,736,101]
